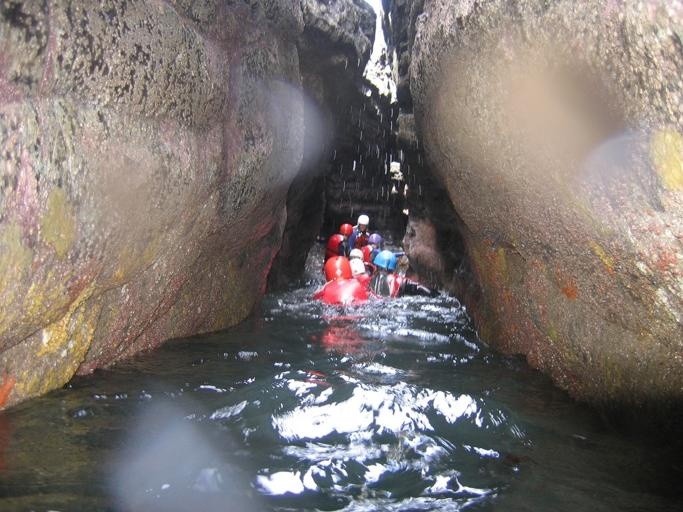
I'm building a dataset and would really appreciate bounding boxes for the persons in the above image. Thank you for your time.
[312,214,433,306]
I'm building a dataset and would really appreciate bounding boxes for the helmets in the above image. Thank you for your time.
[325,215,397,282]
